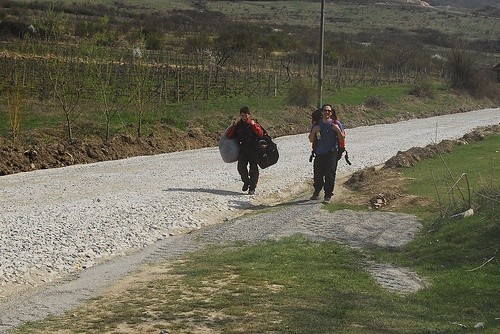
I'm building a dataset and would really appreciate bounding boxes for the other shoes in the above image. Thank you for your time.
[242,179,250,191]
[248,189,254,195]
[324,196,330,204]
[310,194,319,200]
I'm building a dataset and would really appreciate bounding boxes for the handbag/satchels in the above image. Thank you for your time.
[219,122,240,163]
[252,119,279,169]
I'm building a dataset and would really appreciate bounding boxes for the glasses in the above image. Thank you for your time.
[322,109,332,112]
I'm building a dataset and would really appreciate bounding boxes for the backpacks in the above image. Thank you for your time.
[312,107,345,160]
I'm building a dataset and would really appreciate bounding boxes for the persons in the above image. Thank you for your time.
[308,104,346,203]
[226,107,263,196]
